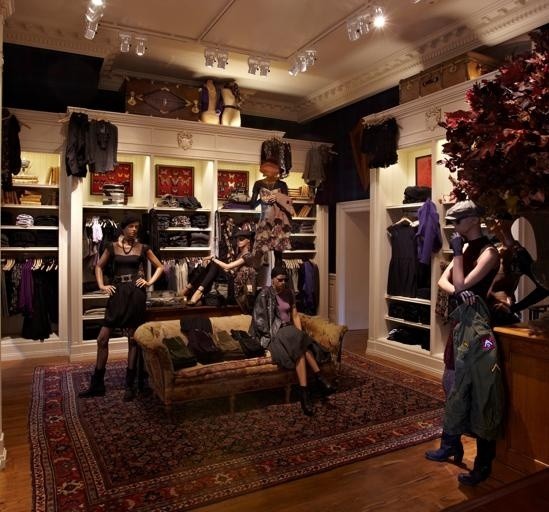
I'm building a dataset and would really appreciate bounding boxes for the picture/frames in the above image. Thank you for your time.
[217,168,250,199]
[155,163,195,197]
[414,154,432,188]
[90,162,134,198]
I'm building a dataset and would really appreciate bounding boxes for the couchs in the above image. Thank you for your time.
[133,313,347,415]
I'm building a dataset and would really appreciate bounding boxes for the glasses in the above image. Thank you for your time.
[455,219,461,225]
[276,277,289,283]
[237,238,246,241]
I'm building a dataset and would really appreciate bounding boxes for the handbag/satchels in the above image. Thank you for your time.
[202,289,225,306]
[163,336,198,370]
[212,329,246,360]
[187,329,224,365]
[157,194,179,207]
[230,329,265,359]
[102,183,128,206]
[175,196,202,210]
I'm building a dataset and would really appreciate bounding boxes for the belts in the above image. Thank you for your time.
[280,321,293,328]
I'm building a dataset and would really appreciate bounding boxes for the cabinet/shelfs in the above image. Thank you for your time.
[152,208,214,250]
[367,144,435,354]
[441,198,457,254]
[1,159,60,249]
[82,207,150,339]
[218,202,316,251]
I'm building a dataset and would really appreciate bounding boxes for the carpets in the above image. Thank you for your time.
[26,353,445,511]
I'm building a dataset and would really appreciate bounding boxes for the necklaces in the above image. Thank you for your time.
[265,177,275,190]
[122,237,135,255]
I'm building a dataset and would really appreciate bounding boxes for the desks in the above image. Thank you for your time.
[493,319,549,473]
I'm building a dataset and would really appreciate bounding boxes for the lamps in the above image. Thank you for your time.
[83,0,105,41]
[287,49,318,75]
[244,54,271,79]
[204,48,230,71]
[118,34,146,56]
[348,7,385,43]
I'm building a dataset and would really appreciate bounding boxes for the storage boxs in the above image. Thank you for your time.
[122,77,203,123]
[396,52,492,103]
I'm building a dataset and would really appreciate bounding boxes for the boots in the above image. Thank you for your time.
[425,431,464,463]
[78,368,107,398]
[300,385,317,416]
[314,370,333,396]
[122,367,137,403]
[458,441,496,486]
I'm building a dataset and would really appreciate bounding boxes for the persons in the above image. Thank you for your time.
[197,77,244,127]
[173,231,254,307]
[424,200,502,486]
[249,156,295,292]
[77,212,165,403]
[441,206,548,438]
[248,264,338,415]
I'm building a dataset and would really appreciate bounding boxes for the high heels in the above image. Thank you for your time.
[174,285,193,301]
[185,288,209,306]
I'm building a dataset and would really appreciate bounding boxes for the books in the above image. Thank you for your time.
[287,184,315,219]
[0,164,60,207]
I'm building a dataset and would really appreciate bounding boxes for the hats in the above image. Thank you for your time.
[271,266,288,278]
[444,200,480,221]
[234,231,251,239]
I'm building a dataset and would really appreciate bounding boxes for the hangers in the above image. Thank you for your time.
[387,211,419,227]
[276,256,303,268]
[93,112,109,122]
[71,108,82,114]
[162,255,213,268]
[3,110,31,133]
[261,130,292,144]
[1,255,59,272]
